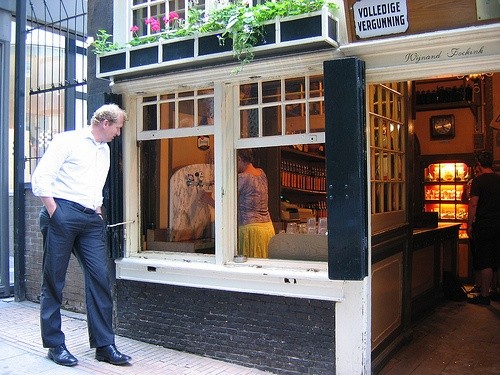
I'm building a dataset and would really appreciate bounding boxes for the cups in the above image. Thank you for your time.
[425,189,463,200]
[286,218,327,233]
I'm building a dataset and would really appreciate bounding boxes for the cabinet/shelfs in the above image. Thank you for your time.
[423,159,471,239]
[254,147,326,234]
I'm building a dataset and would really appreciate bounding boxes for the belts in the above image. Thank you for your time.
[71,203,96,214]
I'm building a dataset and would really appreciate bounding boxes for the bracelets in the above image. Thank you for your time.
[97,213,102,215]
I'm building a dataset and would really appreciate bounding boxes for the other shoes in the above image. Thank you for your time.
[490,292,500,300]
[467,295,491,306]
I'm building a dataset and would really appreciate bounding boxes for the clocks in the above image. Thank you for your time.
[429,114,455,141]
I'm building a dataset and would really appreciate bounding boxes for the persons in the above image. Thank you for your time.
[461,150,500,305]
[200,148,275,259]
[31,103,132,367]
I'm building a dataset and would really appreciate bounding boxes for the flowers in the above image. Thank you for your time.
[96,0,339,51]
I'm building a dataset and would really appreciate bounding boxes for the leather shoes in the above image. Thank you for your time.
[47,343,79,366]
[95,344,133,364]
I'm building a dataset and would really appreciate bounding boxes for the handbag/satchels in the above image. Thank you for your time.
[448,279,467,300]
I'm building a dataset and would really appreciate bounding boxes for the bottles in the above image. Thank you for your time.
[281,160,326,191]
[285,130,324,155]
[428,168,471,181]
[297,200,327,221]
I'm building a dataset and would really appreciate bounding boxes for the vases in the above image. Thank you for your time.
[96,12,340,82]
